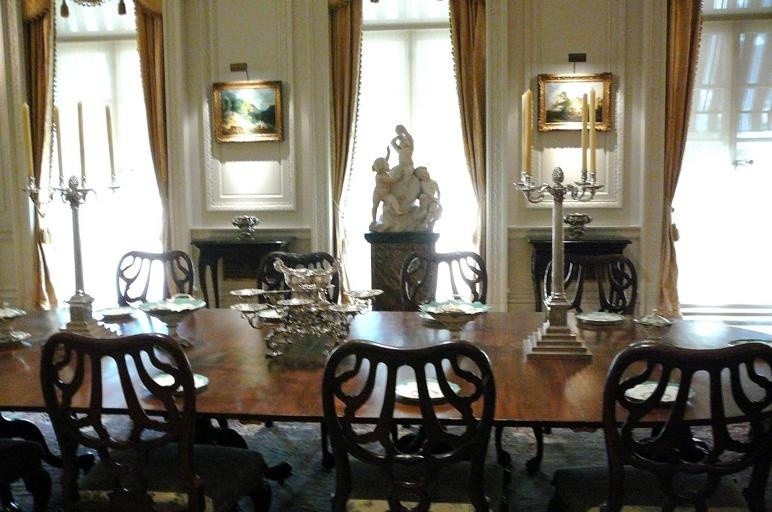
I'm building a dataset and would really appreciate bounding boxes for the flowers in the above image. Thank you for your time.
[230,216,261,226]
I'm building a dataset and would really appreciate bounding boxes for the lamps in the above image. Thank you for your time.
[229,64,252,81]
[567,53,587,73]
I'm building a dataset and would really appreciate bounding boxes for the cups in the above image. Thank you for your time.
[634,308,677,338]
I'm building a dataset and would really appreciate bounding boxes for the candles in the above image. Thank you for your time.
[520,85,600,176]
[18,98,116,179]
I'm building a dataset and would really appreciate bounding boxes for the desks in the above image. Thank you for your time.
[191,237,297,308]
[525,235,633,313]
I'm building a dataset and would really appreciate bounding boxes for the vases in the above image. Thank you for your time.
[236,224,256,241]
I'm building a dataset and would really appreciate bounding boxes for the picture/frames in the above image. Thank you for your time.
[212,80,284,142]
[536,72,613,134]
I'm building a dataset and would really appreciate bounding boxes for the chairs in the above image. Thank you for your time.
[255,250,342,309]
[547,340,771,510]
[321,336,499,511]
[115,251,194,311]
[34,329,270,511]
[540,253,641,317]
[400,250,490,314]
[0,413,99,510]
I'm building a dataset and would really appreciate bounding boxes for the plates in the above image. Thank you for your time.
[94,307,136,320]
[727,338,772,348]
[416,312,441,325]
[624,380,695,405]
[137,372,210,395]
[395,376,461,403]
[575,312,625,326]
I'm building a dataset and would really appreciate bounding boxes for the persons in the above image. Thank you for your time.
[371,144,406,224]
[414,166,443,230]
[390,127,414,200]
